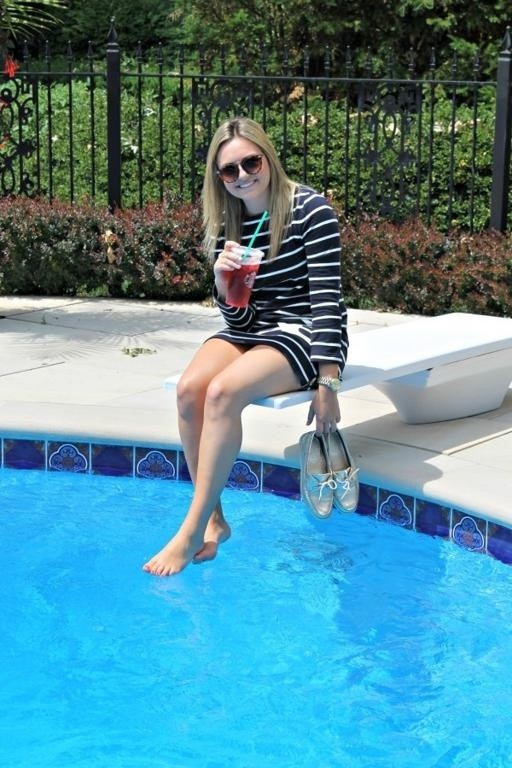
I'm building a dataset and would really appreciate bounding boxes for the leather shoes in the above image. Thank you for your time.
[324,428,360,513]
[299,428,334,518]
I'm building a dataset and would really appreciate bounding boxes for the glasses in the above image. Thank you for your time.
[217,153,265,183]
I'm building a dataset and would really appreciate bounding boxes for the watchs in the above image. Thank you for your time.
[317,376,342,392]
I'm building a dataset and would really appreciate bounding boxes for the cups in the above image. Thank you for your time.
[223,244,264,308]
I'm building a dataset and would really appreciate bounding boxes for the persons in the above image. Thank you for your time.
[142,116,349,576]
